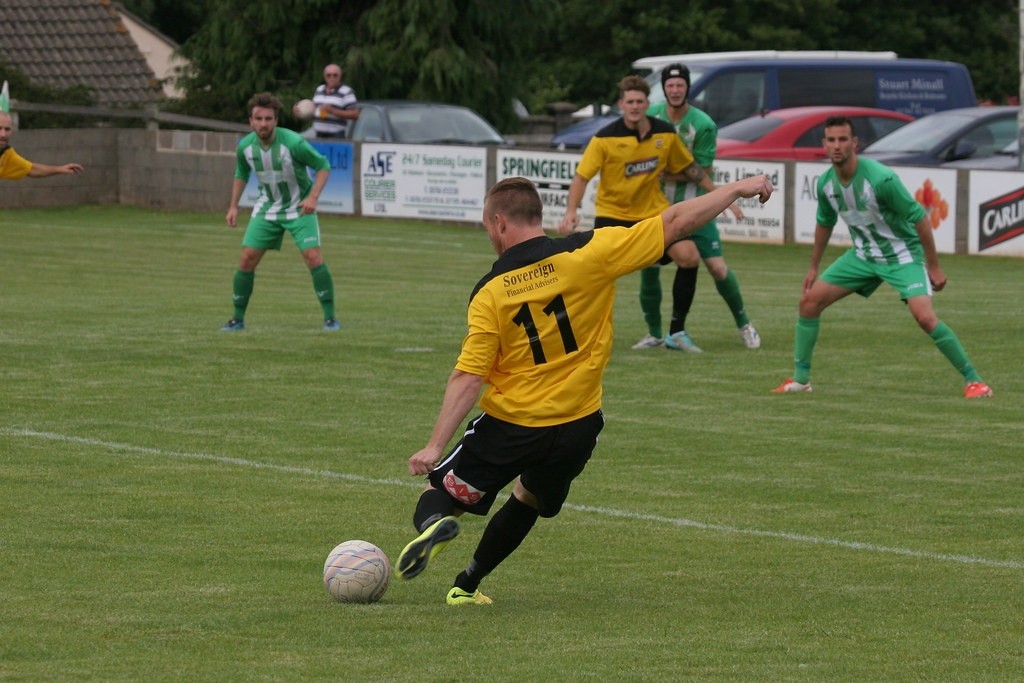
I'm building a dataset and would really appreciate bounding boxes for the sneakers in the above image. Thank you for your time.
[219,318,243,331]
[632,332,665,349]
[738,322,761,349]
[322,317,339,332]
[664,331,701,353]
[446,587,493,606]
[771,378,812,392]
[963,381,993,400]
[393,516,460,581]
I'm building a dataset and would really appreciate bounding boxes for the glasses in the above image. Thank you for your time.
[325,74,339,78]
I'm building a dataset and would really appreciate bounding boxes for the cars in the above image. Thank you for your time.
[942,138,1022,170]
[297,102,519,147]
[714,105,916,159]
[820,104,1020,166]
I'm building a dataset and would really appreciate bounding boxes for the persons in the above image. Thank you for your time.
[219,91,341,330]
[293,63,359,139]
[768,112,993,399]
[0,110,84,179]
[557,74,746,353]
[393,174,774,605]
[631,62,760,349]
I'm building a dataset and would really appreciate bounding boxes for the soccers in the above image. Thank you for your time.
[323,540,392,604]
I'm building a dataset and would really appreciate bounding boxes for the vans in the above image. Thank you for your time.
[549,50,976,151]
